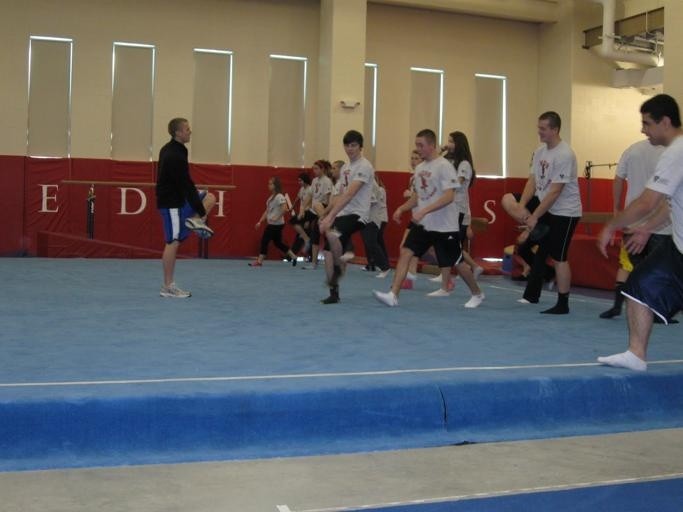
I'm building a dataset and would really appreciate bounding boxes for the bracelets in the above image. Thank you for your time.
[257,222,261,225]
[439,146,446,155]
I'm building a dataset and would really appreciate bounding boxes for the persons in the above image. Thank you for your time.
[317,161,345,261]
[373,179,388,273]
[339,174,391,279]
[248,176,298,267]
[501,110,583,316]
[426,131,485,297]
[288,159,335,271]
[599,135,680,325]
[155,118,216,299]
[371,127,486,310]
[512,232,556,304]
[280,173,318,265]
[399,150,426,283]
[319,130,375,305]
[594,92,683,373]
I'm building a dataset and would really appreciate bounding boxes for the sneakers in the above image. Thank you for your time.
[161,280,192,298]
[186,216,216,241]
